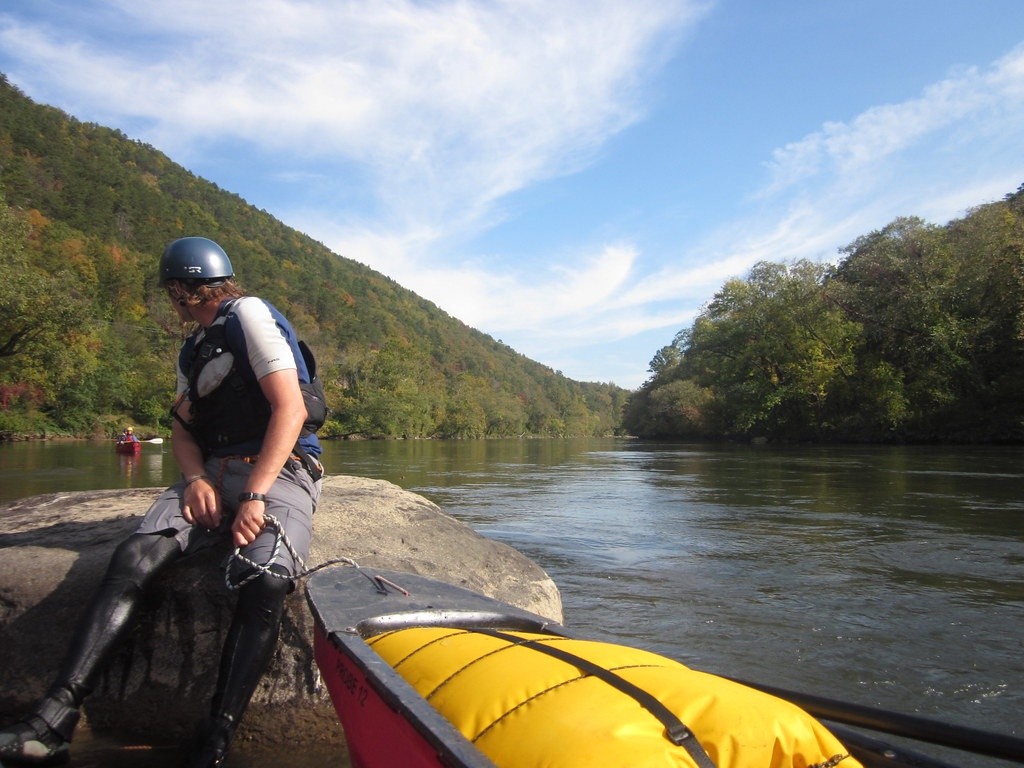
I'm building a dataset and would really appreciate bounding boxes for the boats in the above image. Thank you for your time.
[116,442,140,455]
[304,565,1024,767]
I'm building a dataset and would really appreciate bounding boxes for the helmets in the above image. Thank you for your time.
[127,427,133,432]
[159,237,234,279]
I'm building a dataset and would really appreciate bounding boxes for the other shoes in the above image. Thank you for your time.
[0,718,70,768]
[180,736,226,768]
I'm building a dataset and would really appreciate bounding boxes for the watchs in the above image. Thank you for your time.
[238,490,270,505]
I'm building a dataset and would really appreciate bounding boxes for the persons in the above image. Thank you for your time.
[0,234,327,768]
[119,426,139,443]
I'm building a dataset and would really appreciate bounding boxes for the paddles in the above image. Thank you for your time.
[122,437,164,445]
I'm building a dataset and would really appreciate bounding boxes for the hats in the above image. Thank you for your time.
[124,428,127,431]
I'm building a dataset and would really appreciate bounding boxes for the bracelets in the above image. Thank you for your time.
[182,474,214,487]
